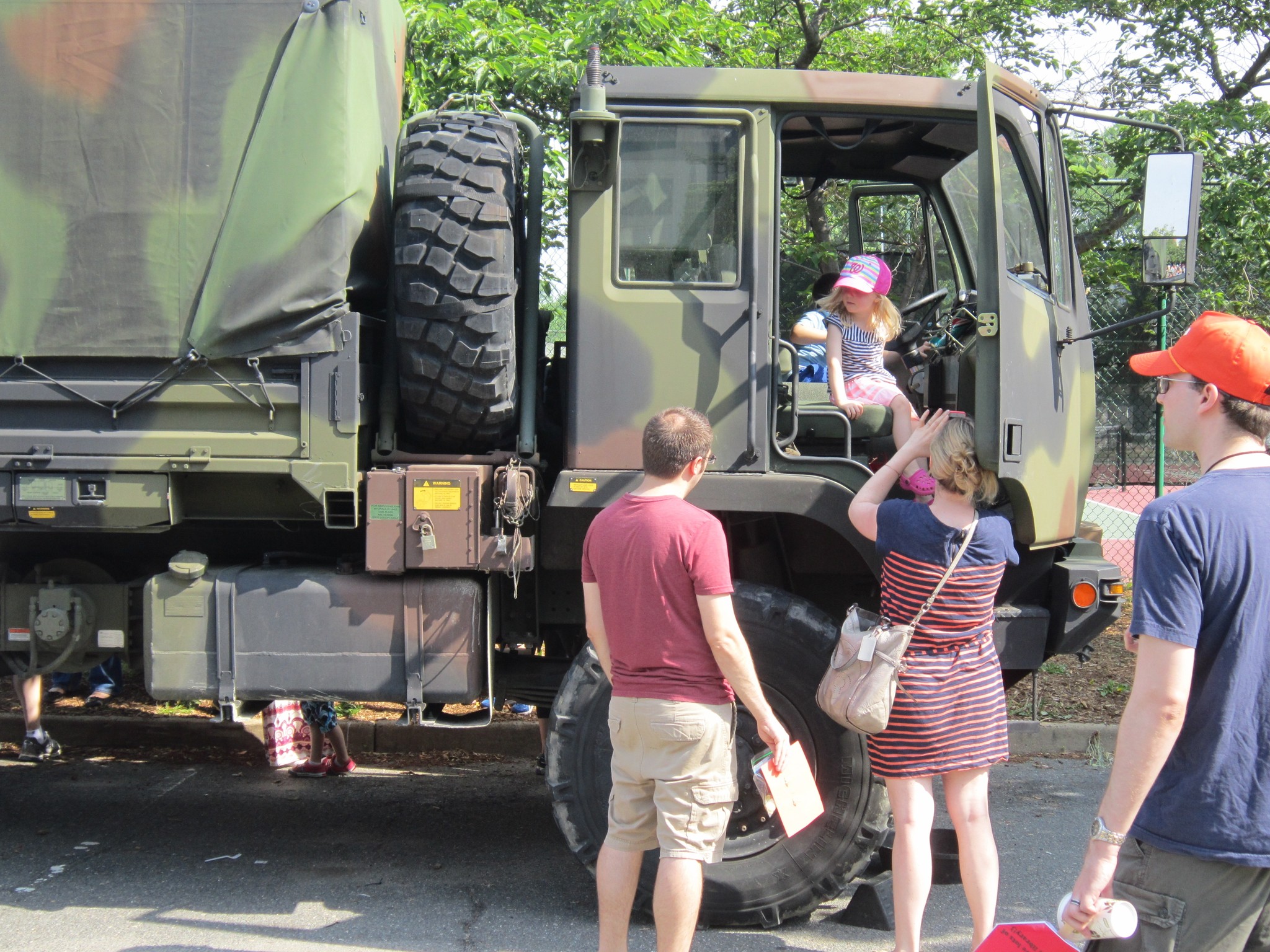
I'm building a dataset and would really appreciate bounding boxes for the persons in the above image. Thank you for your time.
[0,643,561,780]
[581,410,791,950]
[789,271,932,408]
[848,407,1021,950]
[821,253,938,508]
[1058,307,1270,952]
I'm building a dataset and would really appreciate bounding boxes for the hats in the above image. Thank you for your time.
[1130,311,1270,406]
[833,254,892,295]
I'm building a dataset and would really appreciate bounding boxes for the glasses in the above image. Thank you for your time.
[1156,375,1230,398]
[691,453,717,464]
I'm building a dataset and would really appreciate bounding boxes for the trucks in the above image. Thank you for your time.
[0,1,1206,931]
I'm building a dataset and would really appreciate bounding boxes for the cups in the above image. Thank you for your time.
[1057,892,1138,943]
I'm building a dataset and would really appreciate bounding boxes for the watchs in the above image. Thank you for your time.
[1090,817,1126,846]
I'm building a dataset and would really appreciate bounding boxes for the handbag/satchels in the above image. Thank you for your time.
[815,607,915,737]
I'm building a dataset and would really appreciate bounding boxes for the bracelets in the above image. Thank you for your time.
[884,464,901,478]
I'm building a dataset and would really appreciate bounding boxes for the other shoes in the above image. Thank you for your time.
[85,695,115,708]
[43,691,64,703]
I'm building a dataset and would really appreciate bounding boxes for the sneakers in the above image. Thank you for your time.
[535,754,546,776]
[18,731,62,762]
[480,697,507,708]
[320,753,357,774]
[288,759,327,778]
[511,702,534,715]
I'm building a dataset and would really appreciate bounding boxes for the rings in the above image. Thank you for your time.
[1070,899,1080,907]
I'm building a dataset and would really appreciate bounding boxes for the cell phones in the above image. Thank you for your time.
[949,411,965,420]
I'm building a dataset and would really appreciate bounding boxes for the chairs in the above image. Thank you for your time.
[711,241,894,442]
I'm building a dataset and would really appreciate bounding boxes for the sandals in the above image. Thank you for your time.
[899,468,937,496]
